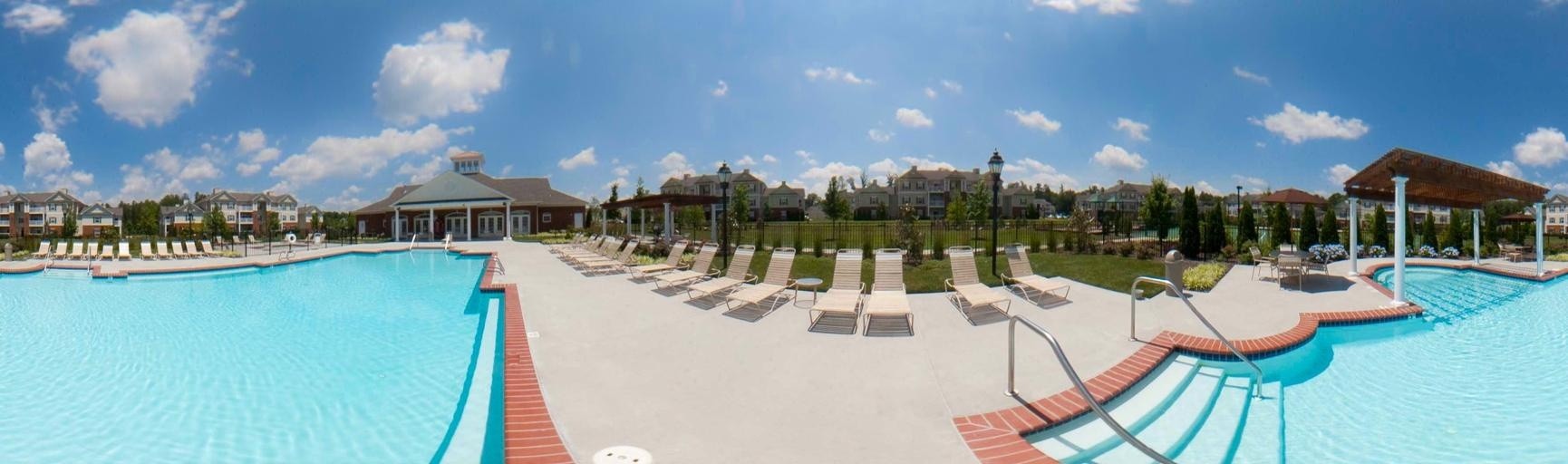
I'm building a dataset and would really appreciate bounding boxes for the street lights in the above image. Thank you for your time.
[717,161,732,267]
[1236,186,1243,219]
[987,148,1004,275]
[185,206,195,239]
[62,207,69,241]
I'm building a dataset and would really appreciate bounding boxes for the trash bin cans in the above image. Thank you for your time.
[4,243,13,261]
[1164,249,1183,297]
[315,233,321,245]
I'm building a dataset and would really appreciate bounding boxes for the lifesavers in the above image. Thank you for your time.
[289,234,296,242]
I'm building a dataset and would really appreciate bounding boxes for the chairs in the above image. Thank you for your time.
[1496,236,1538,261]
[19,231,331,261]
[545,225,1074,340]
[1244,240,1328,297]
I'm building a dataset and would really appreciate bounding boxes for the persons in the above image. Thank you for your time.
[654,223,661,243]
[675,227,679,234]
[435,219,445,236]
